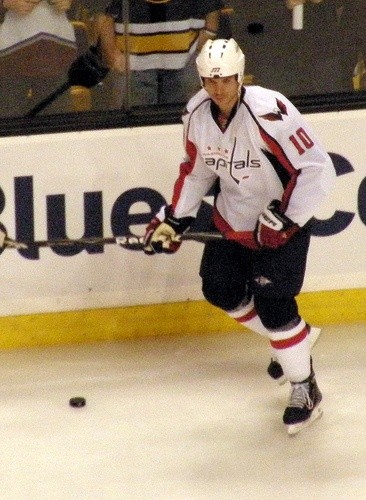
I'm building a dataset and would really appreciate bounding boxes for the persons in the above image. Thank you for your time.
[0,0,233,121]
[142,39,336,435]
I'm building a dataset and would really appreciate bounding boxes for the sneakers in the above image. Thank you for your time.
[282,356,324,435]
[267,323,322,385]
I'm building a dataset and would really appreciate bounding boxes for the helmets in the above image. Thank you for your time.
[196,38,245,98]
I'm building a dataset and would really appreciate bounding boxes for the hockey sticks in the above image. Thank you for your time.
[1,231,258,249]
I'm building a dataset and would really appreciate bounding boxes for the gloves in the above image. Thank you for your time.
[143,205,182,255]
[253,199,298,249]
